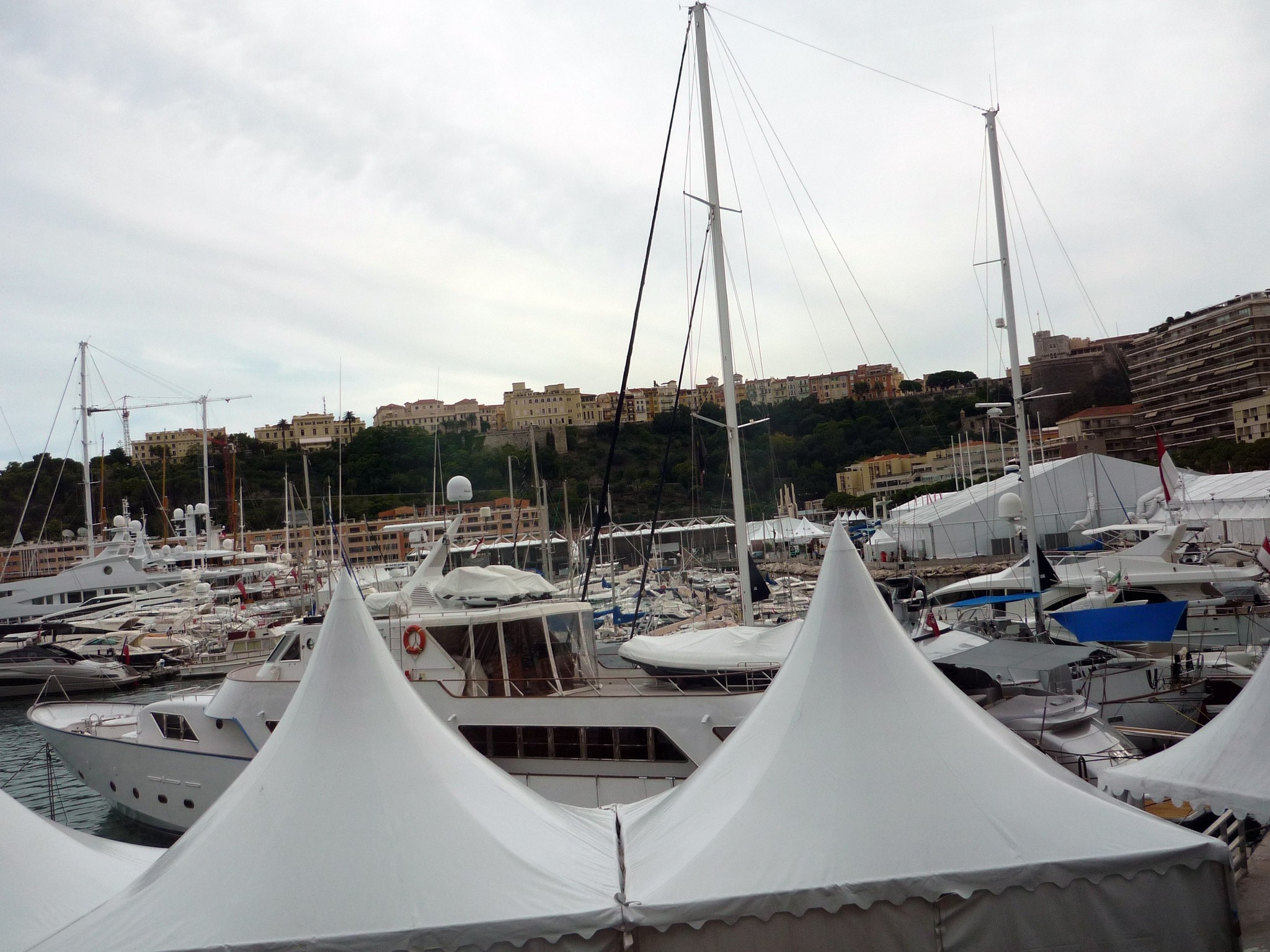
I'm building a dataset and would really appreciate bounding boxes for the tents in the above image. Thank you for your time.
[848,510,857,520]
[856,509,869,520]
[2,788,139,948]
[1090,647,1269,845]
[841,511,849,521]
[881,451,1194,560]
[829,512,842,523]
[620,520,1253,951]
[863,528,898,561]
[1,565,623,950]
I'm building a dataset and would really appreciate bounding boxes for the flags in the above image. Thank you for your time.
[1256,536,1270,573]
[36,631,43,643]
[470,537,484,561]
[304,582,308,592]
[236,579,247,599]
[923,608,941,638]
[219,531,226,539]
[291,570,298,583]
[123,644,130,665]
[1122,572,1131,590]
[269,575,276,588]
[1154,437,1182,504]
[316,574,324,588]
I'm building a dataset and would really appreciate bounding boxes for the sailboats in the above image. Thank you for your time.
[0,0,1269,851]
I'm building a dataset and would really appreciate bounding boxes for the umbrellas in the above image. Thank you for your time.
[791,516,832,539]
[747,521,785,542]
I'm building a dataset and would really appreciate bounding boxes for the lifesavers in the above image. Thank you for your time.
[402,625,426,654]
